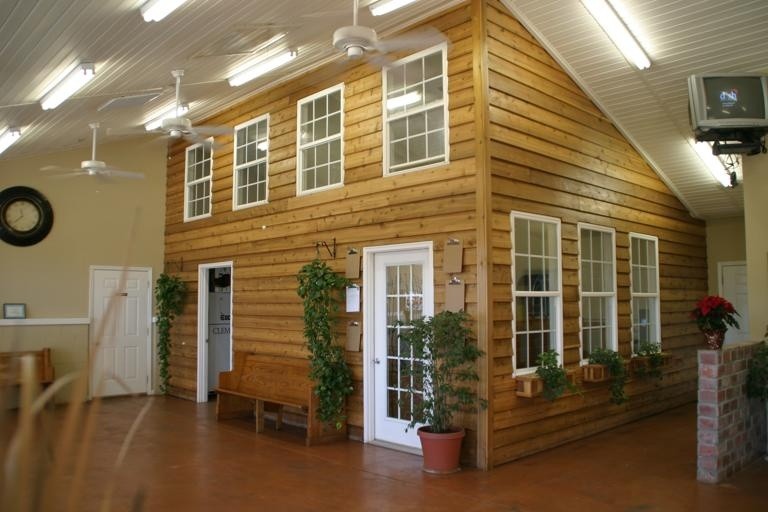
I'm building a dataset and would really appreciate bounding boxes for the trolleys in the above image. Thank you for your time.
[106,69,236,150]
[40,122,147,183]
[259,0,452,96]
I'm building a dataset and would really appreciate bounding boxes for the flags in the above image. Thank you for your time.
[703,329,727,351]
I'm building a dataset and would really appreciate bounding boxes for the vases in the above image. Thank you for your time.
[689,295,742,333]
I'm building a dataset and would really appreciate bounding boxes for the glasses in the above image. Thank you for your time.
[386,91,423,110]
[368,1,416,16]
[580,0,654,72]
[39,62,95,111]
[140,0,186,22]
[257,132,308,152]
[687,131,735,188]
[225,32,298,87]
[136,86,191,132]
[0,125,21,154]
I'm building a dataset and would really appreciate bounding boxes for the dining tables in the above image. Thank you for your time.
[582,346,632,406]
[514,348,587,401]
[389,307,489,476]
[633,341,672,386]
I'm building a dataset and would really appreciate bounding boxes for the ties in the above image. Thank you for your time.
[687,71,768,133]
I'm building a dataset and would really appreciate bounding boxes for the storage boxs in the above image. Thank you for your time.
[213,350,348,446]
[0,347,55,412]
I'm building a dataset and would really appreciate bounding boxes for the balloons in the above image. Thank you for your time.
[0,186,54,246]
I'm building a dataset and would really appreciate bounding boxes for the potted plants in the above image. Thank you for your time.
[3,304,26,319]
